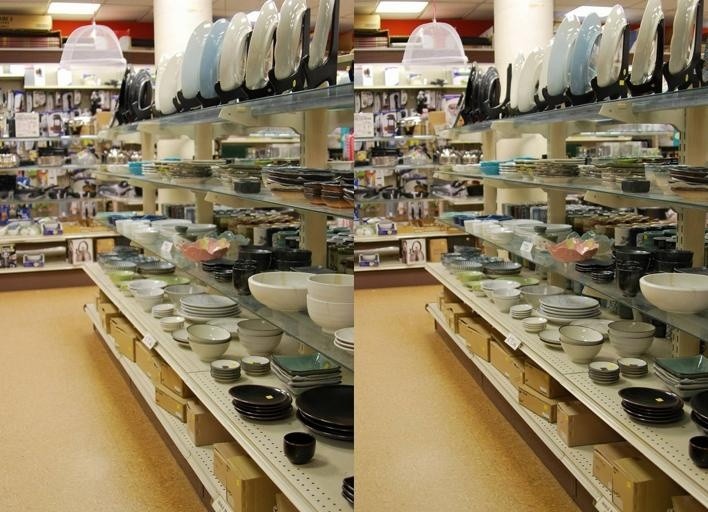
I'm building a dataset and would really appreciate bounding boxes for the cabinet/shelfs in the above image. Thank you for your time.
[72,82,356,507]
[424,82,707,512]
[1,70,154,280]
[354,82,495,277]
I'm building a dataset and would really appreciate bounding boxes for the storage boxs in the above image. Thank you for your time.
[352,14,382,32]
[94,237,116,263]
[0,13,53,37]
[423,234,447,264]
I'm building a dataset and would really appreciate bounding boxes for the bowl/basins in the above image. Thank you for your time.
[465,220,512,248]
[109,212,311,297]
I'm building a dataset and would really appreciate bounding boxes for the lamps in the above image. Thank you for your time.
[401,0,470,66]
[57,0,128,69]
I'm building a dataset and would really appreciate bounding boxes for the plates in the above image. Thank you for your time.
[665,1,696,74]
[118,8,252,126]
[514,217,612,287]
[269,1,308,79]
[436,246,708,474]
[244,0,278,87]
[629,1,667,87]
[451,158,708,192]
[302,1,335,70]
[464,4,625,118]
[103,158,354,210]
[98,245,354,505]
